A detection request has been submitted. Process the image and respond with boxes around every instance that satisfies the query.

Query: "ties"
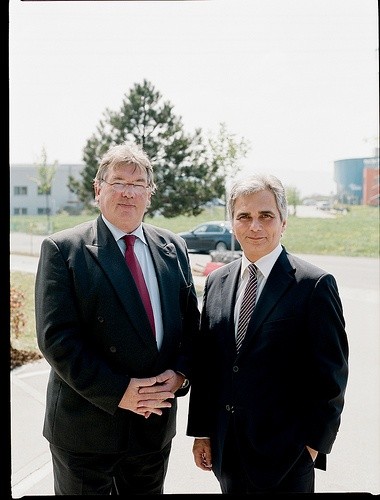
[236,264,258,353]
[120,235,156,337]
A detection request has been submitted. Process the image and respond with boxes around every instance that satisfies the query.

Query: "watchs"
[181,377,190,389]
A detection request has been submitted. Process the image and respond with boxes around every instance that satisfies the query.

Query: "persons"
[193,174,349,493]
[35,145,200,496]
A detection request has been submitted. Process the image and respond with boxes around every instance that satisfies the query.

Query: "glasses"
[98,178,151,192]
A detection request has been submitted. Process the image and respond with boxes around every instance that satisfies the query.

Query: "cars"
[174,221,244,253]
